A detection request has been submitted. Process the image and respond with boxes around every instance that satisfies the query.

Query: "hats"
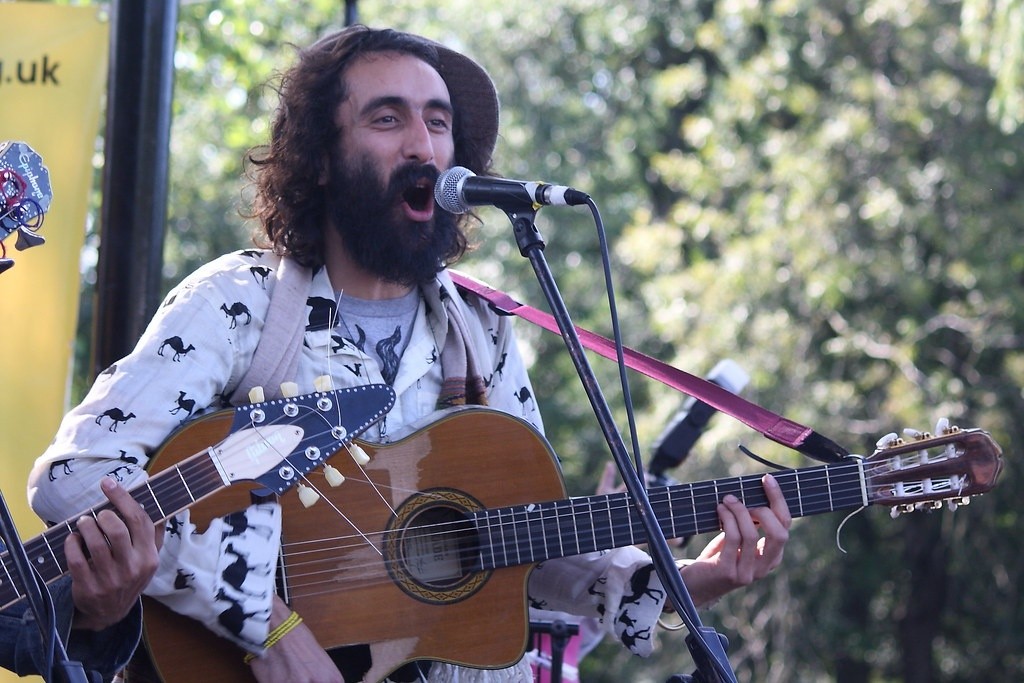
[281,29,498,174]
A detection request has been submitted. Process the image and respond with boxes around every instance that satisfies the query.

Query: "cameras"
[644,359,750,549]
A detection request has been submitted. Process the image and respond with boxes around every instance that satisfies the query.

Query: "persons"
[593,462,684,551]
[27,20,789,683]
[0,473,166,683]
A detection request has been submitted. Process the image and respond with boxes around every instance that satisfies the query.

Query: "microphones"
[434,165,590,215]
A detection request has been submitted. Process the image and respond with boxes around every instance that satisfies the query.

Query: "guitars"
[0,381,398,613]
[140,405,1005,683]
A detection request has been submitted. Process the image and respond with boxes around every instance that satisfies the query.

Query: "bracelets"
[243,611,304,663]
[657,617,686,631]
